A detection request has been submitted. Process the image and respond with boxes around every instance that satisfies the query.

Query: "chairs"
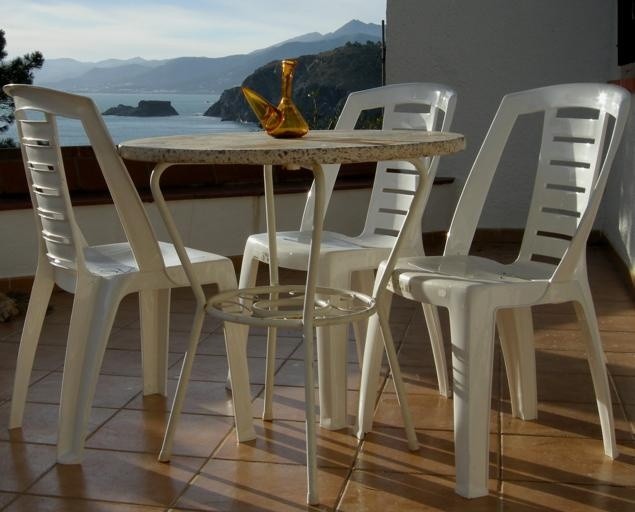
[352,82,633,498]
[224,81,459,429]
[1,82,256,463]
